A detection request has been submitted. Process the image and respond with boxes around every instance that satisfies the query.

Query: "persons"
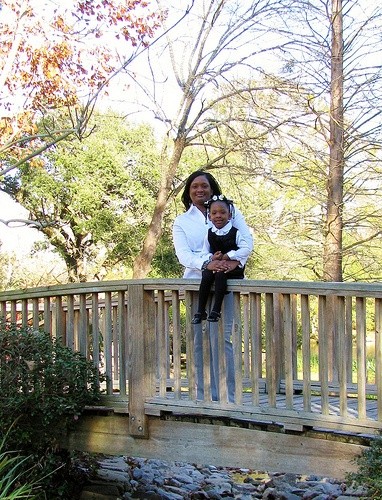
[189,194,250,324]
[173,171,254,403]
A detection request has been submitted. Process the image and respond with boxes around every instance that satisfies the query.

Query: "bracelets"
[202,260,211,269]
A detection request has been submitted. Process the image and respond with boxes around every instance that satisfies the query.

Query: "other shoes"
[190,311,207,324]
[207,310,221,322]
